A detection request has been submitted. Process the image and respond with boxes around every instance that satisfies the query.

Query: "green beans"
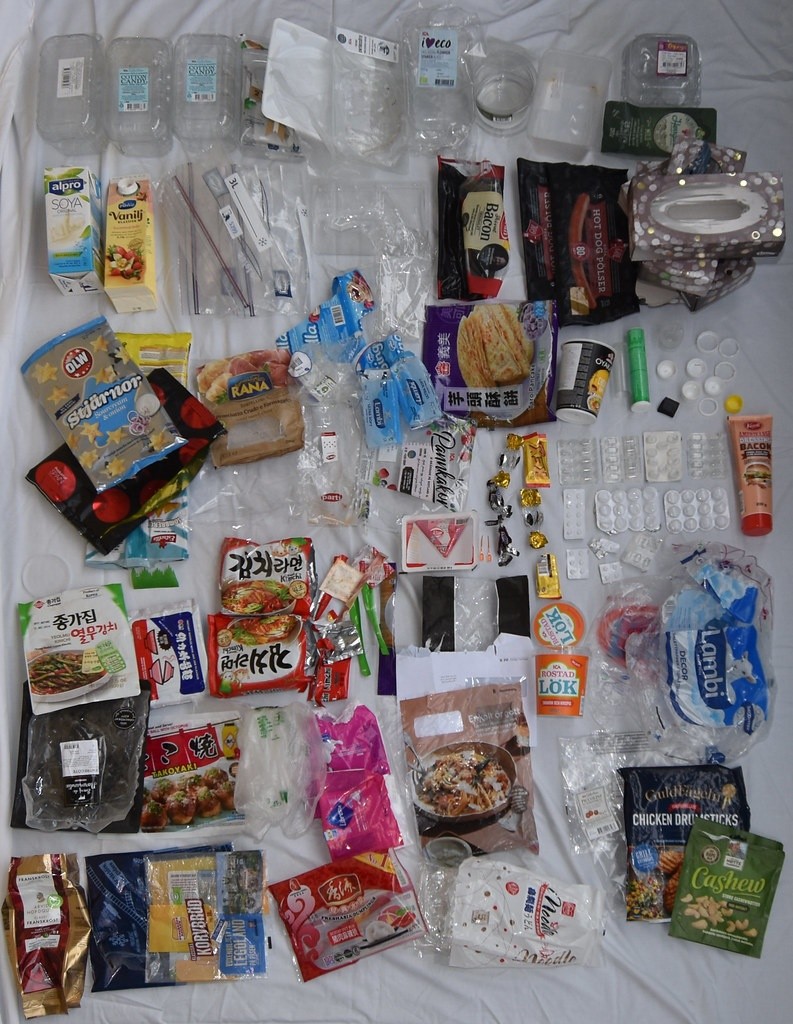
[30,656,94,689]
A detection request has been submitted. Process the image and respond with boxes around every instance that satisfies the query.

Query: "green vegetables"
[232,579,290,644]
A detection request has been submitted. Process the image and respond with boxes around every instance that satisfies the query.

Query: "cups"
[555,338,616,424]
[533,647,590,720]
[473,50,537,132]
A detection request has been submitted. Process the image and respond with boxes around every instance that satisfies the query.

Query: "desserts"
[139,766,238,829]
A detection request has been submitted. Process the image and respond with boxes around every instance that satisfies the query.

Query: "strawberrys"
[108,245,143,276]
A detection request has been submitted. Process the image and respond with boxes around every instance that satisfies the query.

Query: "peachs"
[36,382,221,524]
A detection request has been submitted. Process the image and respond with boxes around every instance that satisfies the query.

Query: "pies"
[457,303,535,389]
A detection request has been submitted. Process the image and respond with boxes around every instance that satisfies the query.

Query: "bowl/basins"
[526,50,612,149]
[101,36,172,156]
[410,741,518,825]
[172,32,240,156]
[36,33,107,155]
[620,33,702,107]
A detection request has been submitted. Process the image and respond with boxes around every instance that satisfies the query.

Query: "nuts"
[681,894,758,937]
